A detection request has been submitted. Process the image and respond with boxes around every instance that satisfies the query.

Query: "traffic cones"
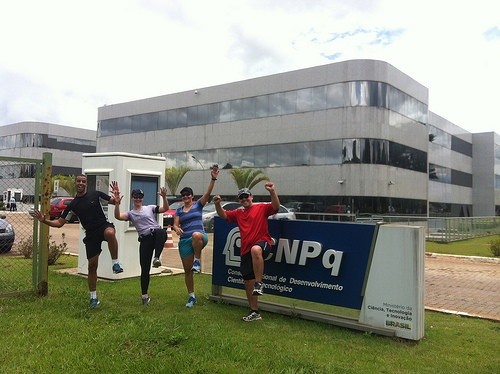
[163,225,174,249]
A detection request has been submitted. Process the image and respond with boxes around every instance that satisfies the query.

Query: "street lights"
[192,156,205,196]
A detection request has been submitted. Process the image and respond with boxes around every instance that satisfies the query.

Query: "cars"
[0,214,15,253]
[164,201,296,234]
[283,202,348,221]
[28,197,79,223]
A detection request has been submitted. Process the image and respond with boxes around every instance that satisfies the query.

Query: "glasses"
[132,195,144,199]
[238,194,249,200]
[180,192,192,197]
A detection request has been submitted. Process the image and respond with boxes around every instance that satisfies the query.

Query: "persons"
[173,167,220,307]
[10,196,16,212]
[213,183,282,322]
[112,187,169,304]
[33,175,124,306]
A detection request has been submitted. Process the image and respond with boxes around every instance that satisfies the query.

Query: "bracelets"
[212,178,217,180]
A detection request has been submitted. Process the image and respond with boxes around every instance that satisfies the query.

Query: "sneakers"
[185,296,197,308]
[90,297,100,310]
[190,260,201,274]
[112,262,124,274]
[152,257,162,269]
[141,296,151,306]
[242,312,262,322]
[251,281,264,296]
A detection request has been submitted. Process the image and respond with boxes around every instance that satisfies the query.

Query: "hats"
[180,186,193,196]
[238,188,252,198]
[131,189,145,198]
